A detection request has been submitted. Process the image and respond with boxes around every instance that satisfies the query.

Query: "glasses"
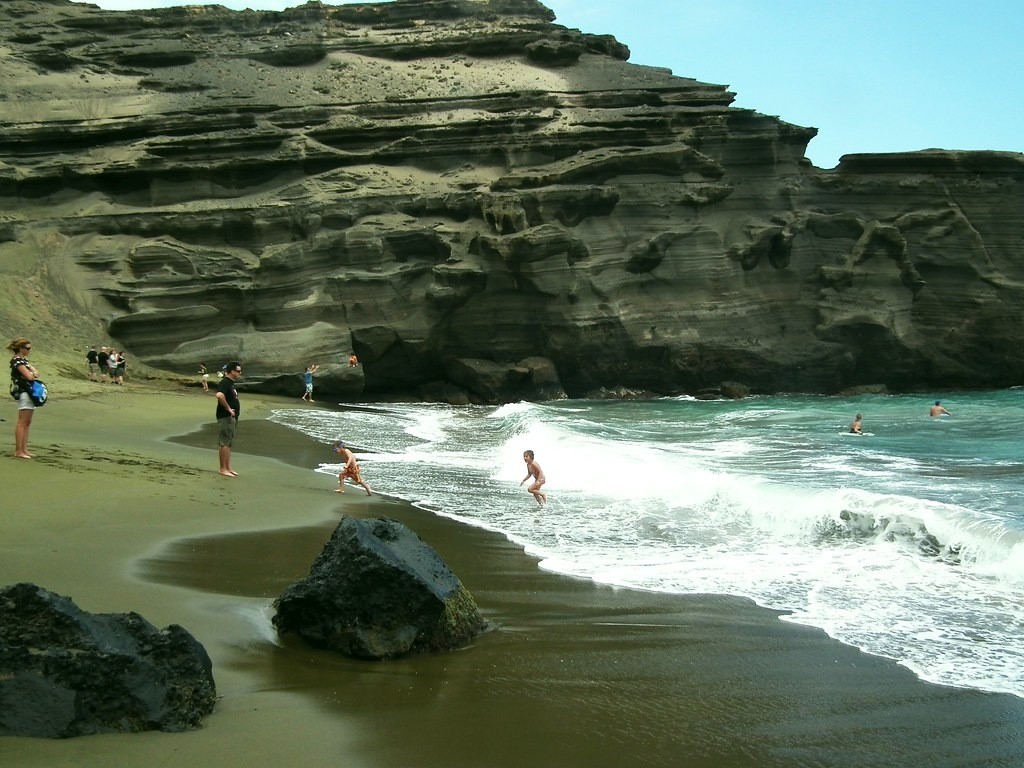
[22,346,31,350]
[234,370,241,373]
[332,448,337,452]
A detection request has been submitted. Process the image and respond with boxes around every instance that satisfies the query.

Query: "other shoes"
[90,378,122,386]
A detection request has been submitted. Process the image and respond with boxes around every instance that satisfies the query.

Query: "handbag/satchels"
[24,379,49,407]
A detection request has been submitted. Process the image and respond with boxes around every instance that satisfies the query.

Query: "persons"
[332,440,371,496]
[98,346,110,383]
[118,351,127,385]
[850,413,864,437]
[518,450,545,506]
[219,365,238,398]
[303,364,319,402]
[198,362,209,392]
[927,400,952,418]
[85,345,100,382]
[6,337,39,460]
[216,362,243,479]
[109,348,118,386]
[347,354,359,367]
